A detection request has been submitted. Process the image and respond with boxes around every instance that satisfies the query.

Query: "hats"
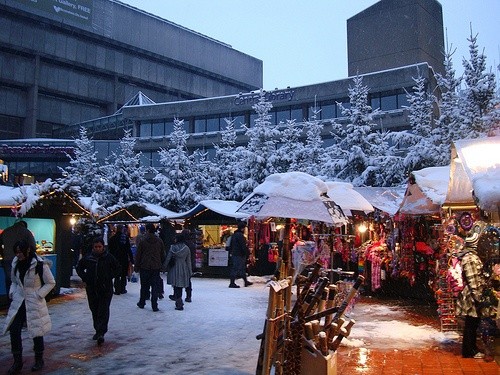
[465,230,479,246]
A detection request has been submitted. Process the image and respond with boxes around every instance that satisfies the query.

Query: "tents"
[0,135,500,226]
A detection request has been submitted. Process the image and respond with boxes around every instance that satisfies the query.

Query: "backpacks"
[445,258,467,298]
[13,260,53,304]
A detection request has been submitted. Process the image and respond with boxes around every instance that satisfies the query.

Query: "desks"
[0,253,59,300]
[203,247,234,276]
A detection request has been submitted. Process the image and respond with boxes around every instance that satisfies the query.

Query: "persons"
[72,225,194,303]
[456,232,487,359]
[76,236,123,346]
[1,239,55,375]
[134,223,165,312]
[0,221,36,308]
[228,223,253,288]
[165,235,193,311]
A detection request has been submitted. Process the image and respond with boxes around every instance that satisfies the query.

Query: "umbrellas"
[235,172,349,277]
[323,182,375,283]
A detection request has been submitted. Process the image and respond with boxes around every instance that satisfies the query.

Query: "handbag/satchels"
[168,256,175,267]
[130,271,139,283]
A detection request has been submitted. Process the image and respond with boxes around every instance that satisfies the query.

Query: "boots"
[185,290,192,302]
[31,351,44,371]
[169,295,176,301]
[8,351,23,374]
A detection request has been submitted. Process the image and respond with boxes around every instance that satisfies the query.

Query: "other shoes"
[474,352,485,359]
[245,281,253,286]
[229,283,240,288]
[96,336,104,345]
